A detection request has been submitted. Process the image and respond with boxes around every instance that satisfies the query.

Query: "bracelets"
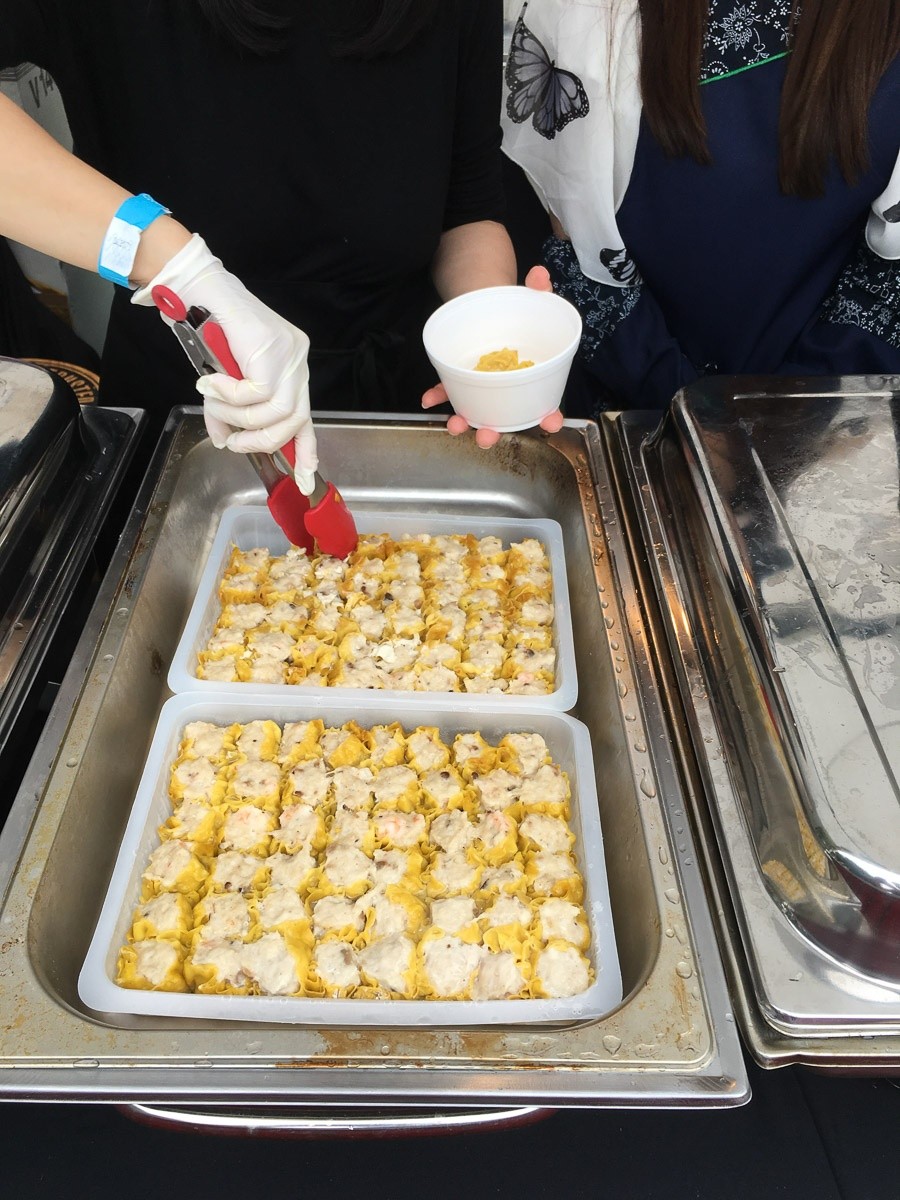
[96,192,171,291]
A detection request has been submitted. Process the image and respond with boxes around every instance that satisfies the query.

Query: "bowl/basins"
[424,285,582,432]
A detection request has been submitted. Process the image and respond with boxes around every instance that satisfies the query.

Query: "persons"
[1,0,564,497]
[492,0,899,413]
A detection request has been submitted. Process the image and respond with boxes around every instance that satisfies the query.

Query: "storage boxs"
[167,506,577,711]
[77,692,622,1024]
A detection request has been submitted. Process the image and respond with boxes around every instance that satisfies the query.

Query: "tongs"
[152,286,361,560]
